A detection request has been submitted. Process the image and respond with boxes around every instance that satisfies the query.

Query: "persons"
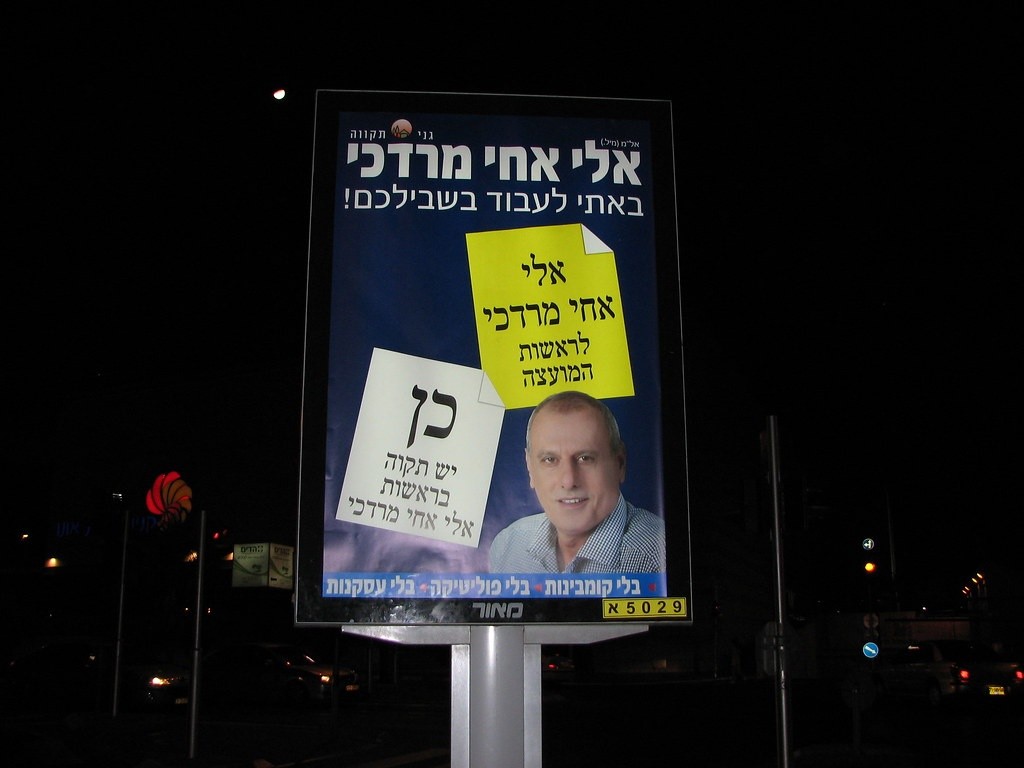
[489,390,666,574]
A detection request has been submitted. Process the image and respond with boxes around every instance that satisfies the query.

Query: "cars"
[843,638,1024,703]
[294,652,361,702]
[542,656,577,682]
[201,643,305,708]
[63,635,191,710]
